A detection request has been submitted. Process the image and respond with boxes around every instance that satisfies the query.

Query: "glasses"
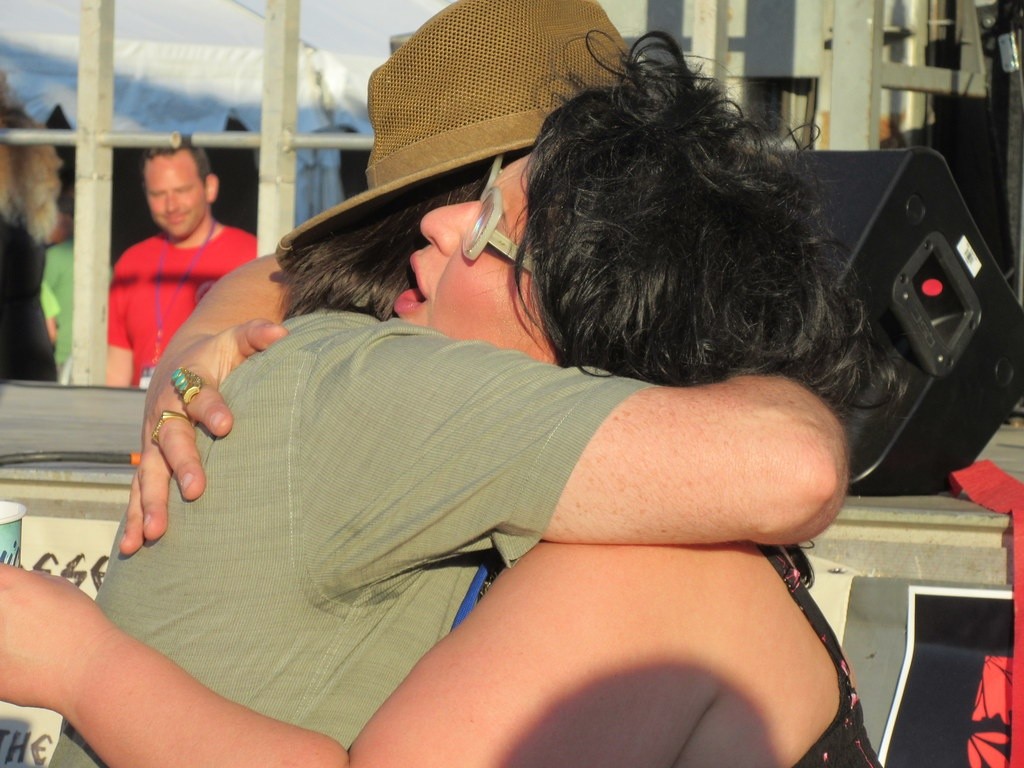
[462,155,541,274]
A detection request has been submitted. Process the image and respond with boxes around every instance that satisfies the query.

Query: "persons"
[1,33,881,767]
[53,0,852,768]
[2,132,258,392]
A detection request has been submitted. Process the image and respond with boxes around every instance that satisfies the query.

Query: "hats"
[275,0,638,272]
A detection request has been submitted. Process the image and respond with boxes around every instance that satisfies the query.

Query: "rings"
[131,452,143,465]
[150,409,191,447]
[170,364,207,407]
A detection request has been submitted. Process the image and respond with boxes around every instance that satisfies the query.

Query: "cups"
[0,501,26,567]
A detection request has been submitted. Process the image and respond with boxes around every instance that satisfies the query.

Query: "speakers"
[761,145,1024,496]
